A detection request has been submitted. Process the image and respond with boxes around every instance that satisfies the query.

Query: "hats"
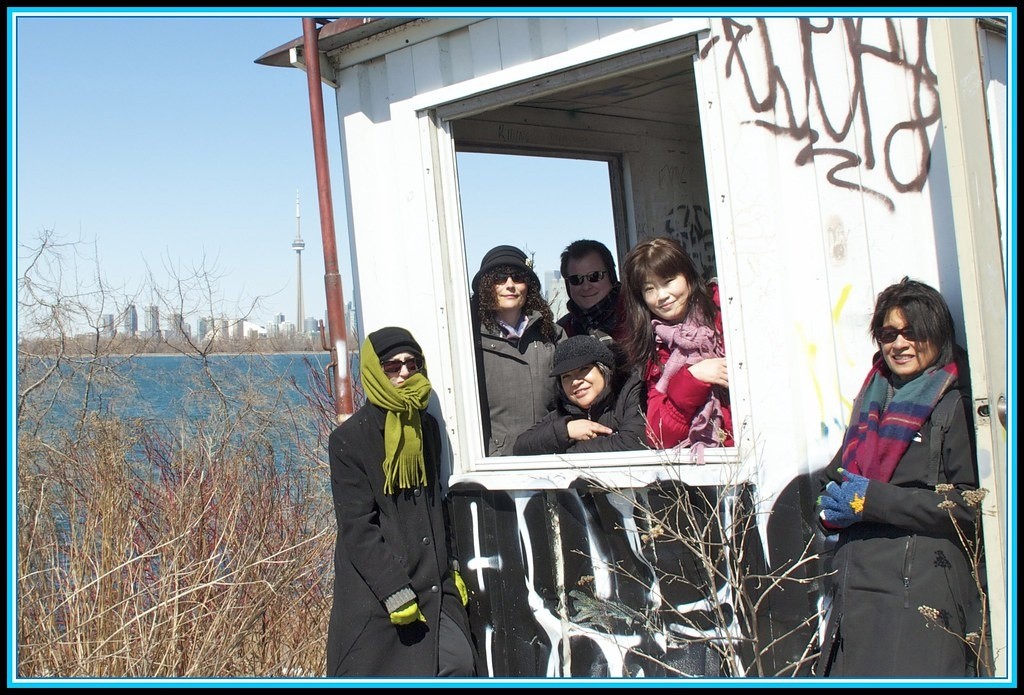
[471,245,541,291]
[548,334,613,378]
[367,327,422,362]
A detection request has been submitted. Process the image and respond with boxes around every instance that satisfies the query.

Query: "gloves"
[390,599,427,625]
[816,468,869,531]
[454,570,469,609]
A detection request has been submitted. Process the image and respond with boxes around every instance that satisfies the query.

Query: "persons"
[513,334,651,456]
[613,234,737,447]
[552,238,647,380]
[468,245,569,457]
[801,273,986,681]
[325,326,488,678]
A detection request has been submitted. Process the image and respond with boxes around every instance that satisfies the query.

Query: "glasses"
[876,324,927,343]
[563,268,610,286]
[380,356,424,373]
[492,270,527,285]
